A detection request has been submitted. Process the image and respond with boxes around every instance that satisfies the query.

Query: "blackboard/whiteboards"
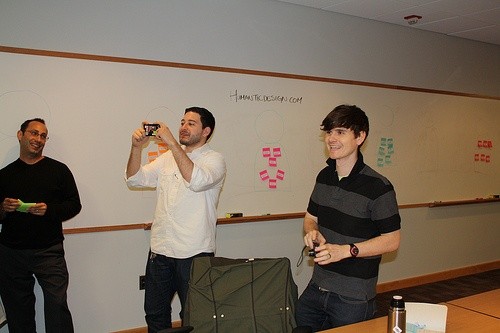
[0,44,496,234]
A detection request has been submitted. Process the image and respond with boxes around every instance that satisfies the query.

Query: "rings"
[35,209,39,213]
[328,254,331,258]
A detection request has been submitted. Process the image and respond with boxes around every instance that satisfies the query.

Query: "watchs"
[349,243,359,258]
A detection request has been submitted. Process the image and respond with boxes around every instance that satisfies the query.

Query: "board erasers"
[226,212,243,218]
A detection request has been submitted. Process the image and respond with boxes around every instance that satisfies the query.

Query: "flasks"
[387,296,406,333]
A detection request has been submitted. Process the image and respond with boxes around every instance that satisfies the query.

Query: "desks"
[316,287,500,333]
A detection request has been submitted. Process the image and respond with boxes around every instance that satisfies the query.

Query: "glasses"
[25,130,49,142]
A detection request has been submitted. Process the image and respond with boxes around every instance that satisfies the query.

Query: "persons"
[0,118,82,333]
[291,104,401,333]
[124,107,227,333]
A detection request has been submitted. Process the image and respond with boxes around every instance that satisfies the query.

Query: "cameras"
[144,123,161,136]
[308,241,320,257]
